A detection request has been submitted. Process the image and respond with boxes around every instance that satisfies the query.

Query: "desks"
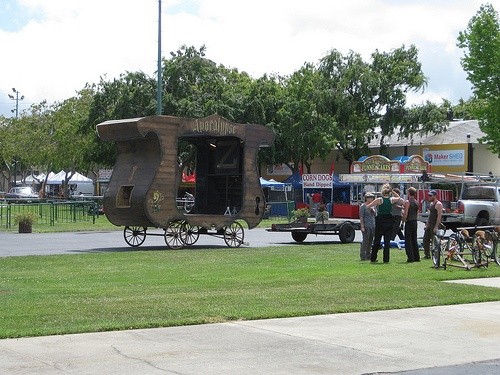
[207,173,242,214]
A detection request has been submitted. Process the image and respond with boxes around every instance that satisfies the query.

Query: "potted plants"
[290,207,310,222]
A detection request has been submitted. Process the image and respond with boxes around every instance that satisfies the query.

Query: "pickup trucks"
[418,184,500,236]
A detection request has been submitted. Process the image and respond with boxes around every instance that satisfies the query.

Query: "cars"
[6,186,40,204]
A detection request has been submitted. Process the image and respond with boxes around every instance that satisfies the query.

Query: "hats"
[364,192,375,198]
[427,190,438,196]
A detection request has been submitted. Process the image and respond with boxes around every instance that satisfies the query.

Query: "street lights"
[8,88,25,186]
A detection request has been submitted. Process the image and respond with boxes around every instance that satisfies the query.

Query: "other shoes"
[359,255,431,263]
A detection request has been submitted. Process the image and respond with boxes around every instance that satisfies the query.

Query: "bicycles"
[431,221,500,270]
